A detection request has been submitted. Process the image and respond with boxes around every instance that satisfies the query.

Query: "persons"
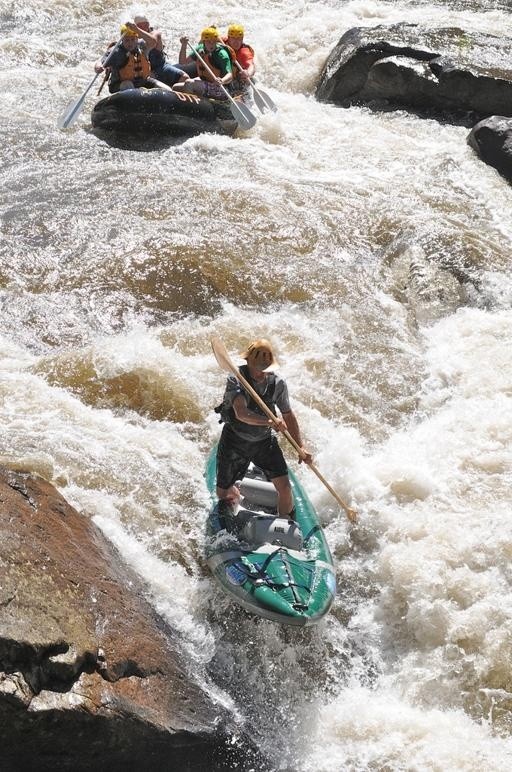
[94,15,255,103]
[216,339,313,523]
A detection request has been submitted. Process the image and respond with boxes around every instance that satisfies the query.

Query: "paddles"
[209,333,358,522]
[59,26,132,130]
[217,37,278,116]
[185,40,257,131]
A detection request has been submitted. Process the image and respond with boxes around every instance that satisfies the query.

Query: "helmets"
[198,28,221,45]
[240,338,280,372]
[121,25,138,37]
[228,25,245,39]
[134,13,148,25]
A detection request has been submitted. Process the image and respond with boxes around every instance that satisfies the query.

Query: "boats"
[202,437,336,627]
[91,85,256,152]
